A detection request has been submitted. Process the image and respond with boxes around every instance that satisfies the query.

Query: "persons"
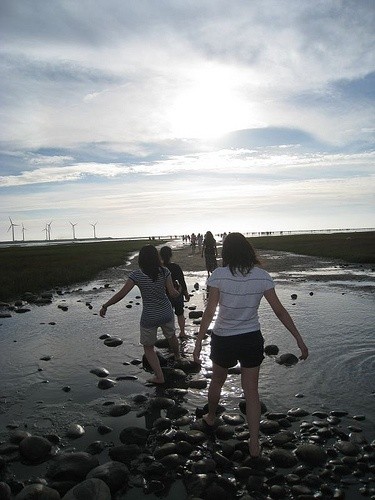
[200,231,219,278]
[191,231,310,460]
[148,231,232,256]
[158,246,191,353]
[99,244,187,386]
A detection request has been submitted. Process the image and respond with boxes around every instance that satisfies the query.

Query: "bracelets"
[102,305,107,309]
[195,343,201,347]
